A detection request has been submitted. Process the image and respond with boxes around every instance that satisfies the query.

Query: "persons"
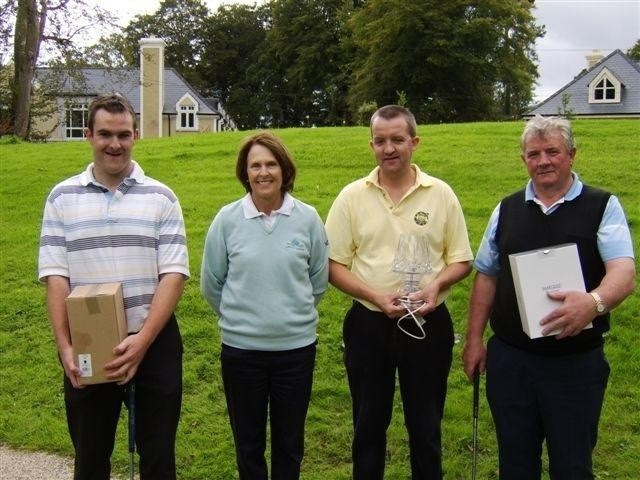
[315,102,473,478]
[196,132,330,479]
[33,90,193,478]
[450,112,638,480]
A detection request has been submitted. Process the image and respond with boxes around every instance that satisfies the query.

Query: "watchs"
[587,290,608,317]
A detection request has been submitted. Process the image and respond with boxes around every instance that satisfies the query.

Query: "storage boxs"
[63,282,129,387]
